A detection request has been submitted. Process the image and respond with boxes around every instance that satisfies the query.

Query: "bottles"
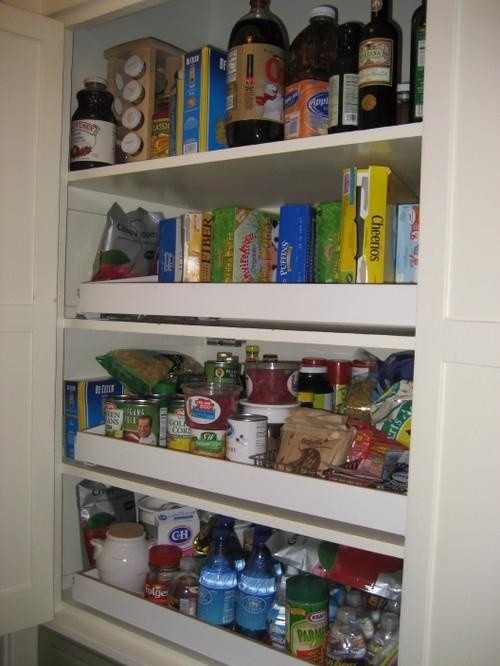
[326,20,365,134]
[357,0,399,131]
[283,5,341,140]
[68,76,118,172]
[408,0,428,124]
[143,513,401,666]
[216,344,376,415]
[225,0,288,148]
[114,56,169,154]
[396,83,411,125]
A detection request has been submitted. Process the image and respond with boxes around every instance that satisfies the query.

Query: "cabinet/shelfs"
[0,0,500,666]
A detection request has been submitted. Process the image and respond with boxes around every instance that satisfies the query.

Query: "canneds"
[225,415,268,465]
[149,393,171,447]
[204,360,238,389]
[122,400,160,443]
[166,397,192,451]
[103,392,134,438]
